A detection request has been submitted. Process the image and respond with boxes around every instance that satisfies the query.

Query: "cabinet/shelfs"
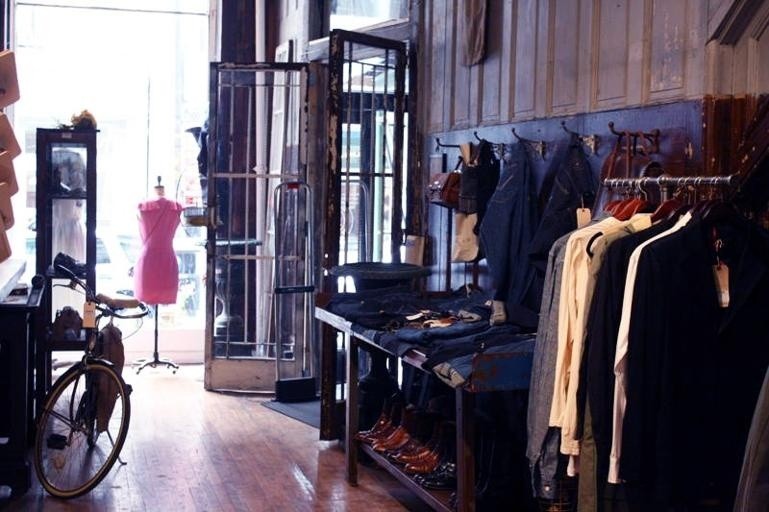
[314,305,475,512]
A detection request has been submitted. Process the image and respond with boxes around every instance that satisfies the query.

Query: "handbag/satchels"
[54,307,82,341]
[589,151,665,222]
[451,213,479,262]
[427,173,460,208]
[458,160,500,213]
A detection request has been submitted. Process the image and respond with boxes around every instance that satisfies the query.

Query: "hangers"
[603,175,754,235]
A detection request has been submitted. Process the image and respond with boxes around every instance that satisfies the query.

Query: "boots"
[354,390,497,512]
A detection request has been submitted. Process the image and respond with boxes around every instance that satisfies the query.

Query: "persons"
[52,150,87,264]
[135,185,186,305]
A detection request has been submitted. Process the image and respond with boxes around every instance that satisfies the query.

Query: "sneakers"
[54,253,87,277]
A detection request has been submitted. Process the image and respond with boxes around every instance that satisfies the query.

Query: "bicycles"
[35,264,151,501]
